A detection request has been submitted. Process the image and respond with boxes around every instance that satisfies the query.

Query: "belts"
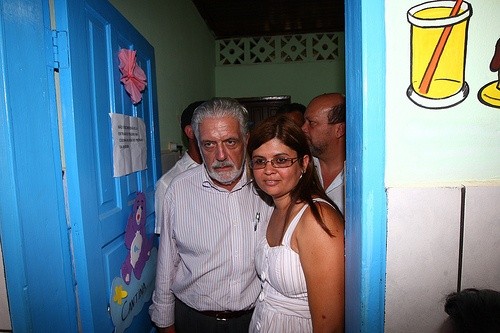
[193,308,254,320]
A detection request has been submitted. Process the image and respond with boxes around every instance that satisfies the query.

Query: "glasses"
[248,156,299,168]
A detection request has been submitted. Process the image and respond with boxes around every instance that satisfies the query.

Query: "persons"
[246,115,345,333]
[300,93,346,218]
[273,103,307,127]
[154,101,206,234]
[146,96,275,333]
